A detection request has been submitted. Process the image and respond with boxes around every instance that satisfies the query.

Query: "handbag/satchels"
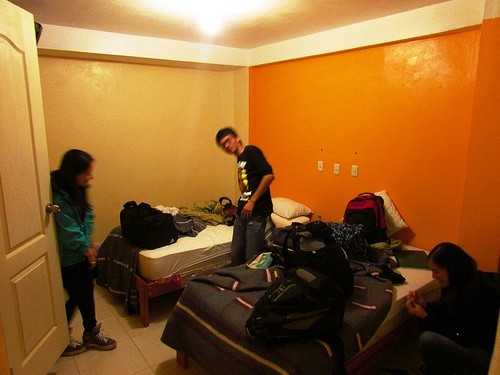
[219,197,238,225]
[282,221,372,301]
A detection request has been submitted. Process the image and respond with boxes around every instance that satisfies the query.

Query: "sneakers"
[60,327,86,356]
[83,322,117,350]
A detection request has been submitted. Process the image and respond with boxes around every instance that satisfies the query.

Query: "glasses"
[220,136,234,149]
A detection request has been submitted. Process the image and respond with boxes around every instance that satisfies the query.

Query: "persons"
[406,241,500,375]
[214,127,277,270]
[49,148,117,357]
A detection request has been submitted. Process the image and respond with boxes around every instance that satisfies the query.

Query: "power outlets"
[334,164,339,174]
[317,161,323,171]
[352,165,359,176]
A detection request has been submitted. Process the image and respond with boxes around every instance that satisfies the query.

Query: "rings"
[90,261,95,264]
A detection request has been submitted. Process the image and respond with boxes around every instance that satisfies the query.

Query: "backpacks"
[120,201,178,248]
[244,264,345,346]
[343,193,388,243]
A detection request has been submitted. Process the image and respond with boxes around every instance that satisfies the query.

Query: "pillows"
[341,190,407,237]
[271,196,311,227]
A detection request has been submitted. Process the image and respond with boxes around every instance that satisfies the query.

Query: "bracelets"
[250,198,255,203]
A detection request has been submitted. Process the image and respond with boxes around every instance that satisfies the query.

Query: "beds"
[133,225,442,375]
